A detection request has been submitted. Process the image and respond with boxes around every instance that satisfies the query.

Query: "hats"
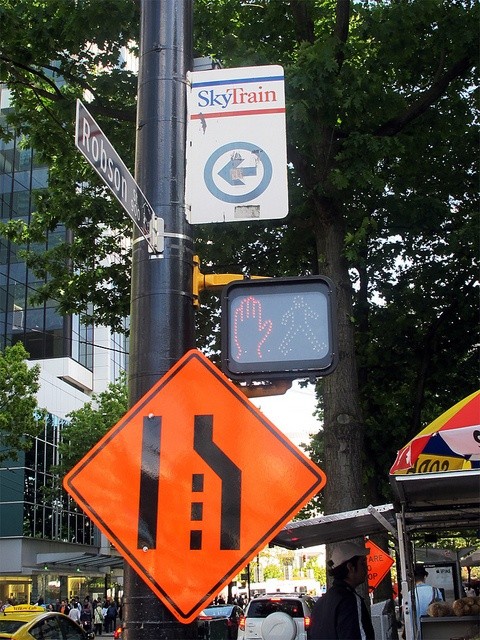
[414,564,428,578]
[329,542,370,570]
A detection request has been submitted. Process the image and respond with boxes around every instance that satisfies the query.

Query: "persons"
[34,599,46,610]
[94,603,102,634]
[97,597,101,603]
[54,599,60,612]
[209,591,251,606]
[70,599,81,611]
[409,565,443,639]
[74,596,81,603]
[102,603,109,632]
[82,603,92,632]
[58,601,69,614]
[308,539,375,639]
[47,604,54,611]
[68,604,80,624]
[107,603,117,632]
[84,599,90,603]
[65,600,70,609]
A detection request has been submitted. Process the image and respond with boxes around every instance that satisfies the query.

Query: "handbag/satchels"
[96,607,103,622]
[428,586,442,606]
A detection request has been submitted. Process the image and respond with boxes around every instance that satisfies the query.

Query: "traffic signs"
[74,99,162,253]
[184,65,289,224]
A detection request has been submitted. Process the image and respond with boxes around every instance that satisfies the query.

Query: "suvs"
[237,593,316,640]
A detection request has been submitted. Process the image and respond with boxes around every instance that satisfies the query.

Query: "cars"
[0,604,95,640]
[198,604,245,640]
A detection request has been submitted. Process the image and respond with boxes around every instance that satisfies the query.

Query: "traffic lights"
[222,275,339,380]
